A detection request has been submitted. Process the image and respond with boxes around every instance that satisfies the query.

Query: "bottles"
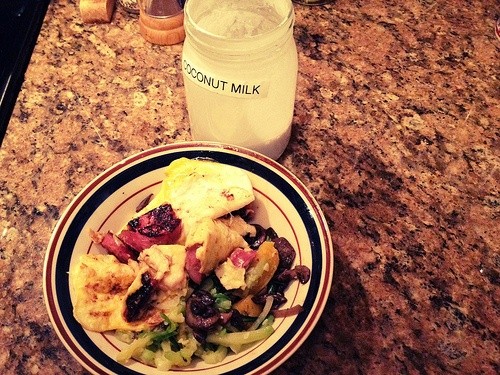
[180,0,299,161]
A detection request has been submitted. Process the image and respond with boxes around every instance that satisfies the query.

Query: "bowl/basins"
[42,140,336,375]
